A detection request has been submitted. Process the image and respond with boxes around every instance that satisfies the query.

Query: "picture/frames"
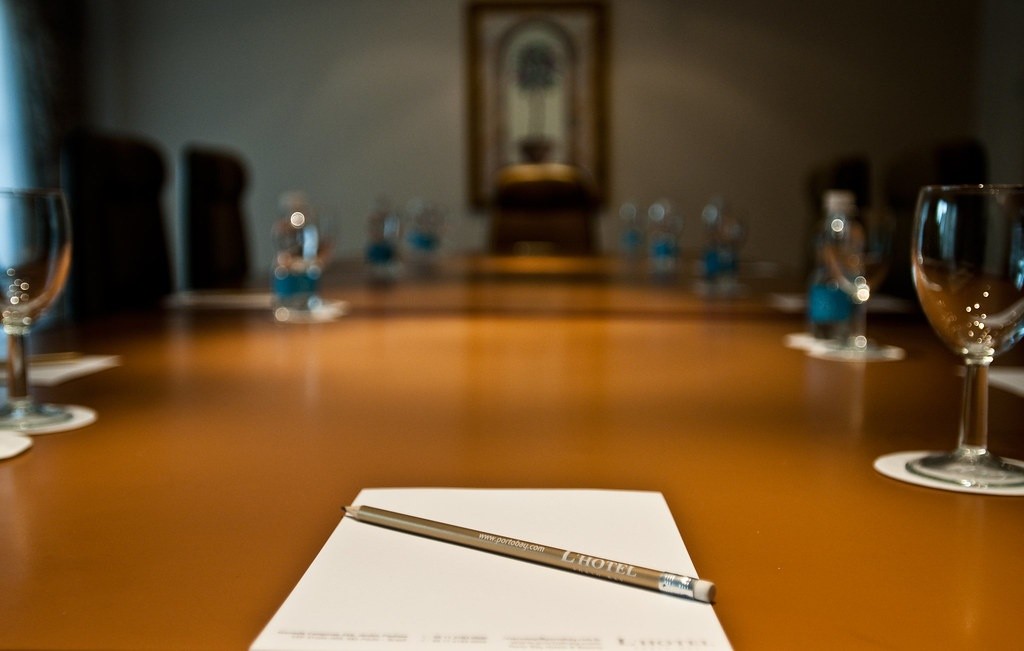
[459,0,618,212]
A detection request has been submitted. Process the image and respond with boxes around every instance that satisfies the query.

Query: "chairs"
[45,126,178,323]
[182,139,254,294]
[798,129,993,328]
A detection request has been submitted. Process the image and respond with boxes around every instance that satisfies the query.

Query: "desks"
[0,253,1024,651]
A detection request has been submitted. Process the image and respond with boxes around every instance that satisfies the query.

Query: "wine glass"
[908,181,1024,488]
[0,185,73,429]
[818,198,906,363]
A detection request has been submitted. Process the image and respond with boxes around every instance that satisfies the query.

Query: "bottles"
[808,189,862,340]
[365,188,402,288]
[270,187,320,318]
[409,201,442,276]
[621,195,741,297]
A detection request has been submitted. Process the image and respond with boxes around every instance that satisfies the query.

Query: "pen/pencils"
[339,504,717,603]
